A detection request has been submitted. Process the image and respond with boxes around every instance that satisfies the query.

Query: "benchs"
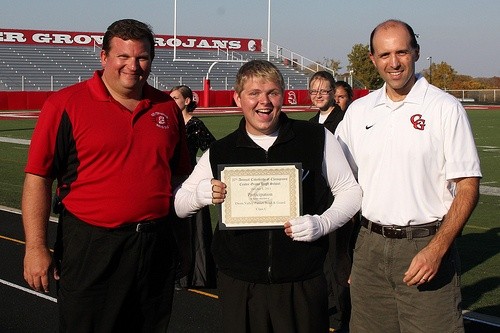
[0,43,310,91]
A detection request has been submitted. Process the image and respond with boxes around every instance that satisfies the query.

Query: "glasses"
[309,89,332,95]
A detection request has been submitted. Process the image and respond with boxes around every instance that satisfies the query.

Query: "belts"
[118,215,174,232]
[360,214,443,239]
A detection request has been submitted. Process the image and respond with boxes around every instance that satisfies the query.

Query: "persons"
[169,85,217,290]
[334,80,353,111]
[308,70,344,135]
[173,59,363,333]
[333,19,500,333]
[21,19,186,333]
